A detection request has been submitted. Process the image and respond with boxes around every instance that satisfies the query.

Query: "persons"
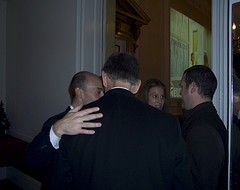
[25,70,105,190]
[61,52,196,190]
[179,65,229,190]
[137,78,167,113]
[230,74,240,190]
